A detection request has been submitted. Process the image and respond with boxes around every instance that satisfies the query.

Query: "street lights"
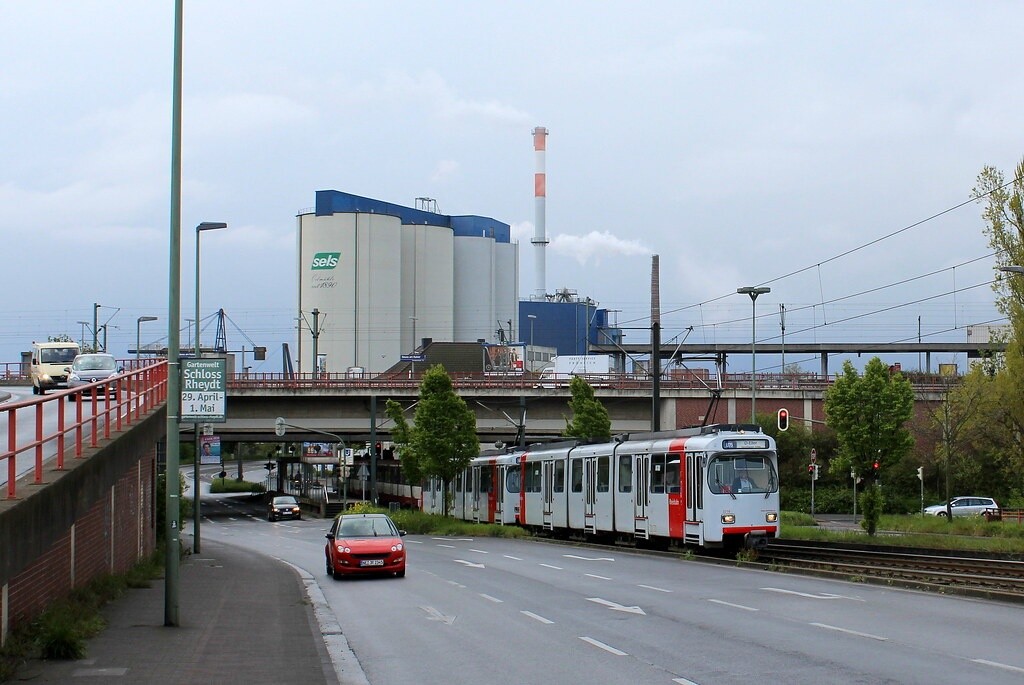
[737,286,771,426]
[193,221,228,550]
[527,314,536,370]
[136,316,158,358]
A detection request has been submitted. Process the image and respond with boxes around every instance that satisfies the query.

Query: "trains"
[349,423,782,555]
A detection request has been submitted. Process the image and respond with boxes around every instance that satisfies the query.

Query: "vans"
[30,342,81,395]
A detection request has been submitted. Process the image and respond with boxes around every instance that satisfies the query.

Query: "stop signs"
[810,447,817,463]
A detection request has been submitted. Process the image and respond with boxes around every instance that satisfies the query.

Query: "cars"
[267,496,302,522]
[920,496,1000,518]
[64,353,125,402]
[325,513,407,580]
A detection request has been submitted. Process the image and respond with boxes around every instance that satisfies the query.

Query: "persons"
[511,348,517,362]
[295,471,302,480]
[314,444,321,455]
[732,470,757,493]
[203,443,213,456]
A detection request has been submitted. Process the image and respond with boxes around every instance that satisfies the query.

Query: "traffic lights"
[779,410,787,429]
[272,463,276,470]
[916,468,922,480]
[376,443,381,454]
[809,465,814,476]
[874,462,879,468]
[264,463,270,470]
